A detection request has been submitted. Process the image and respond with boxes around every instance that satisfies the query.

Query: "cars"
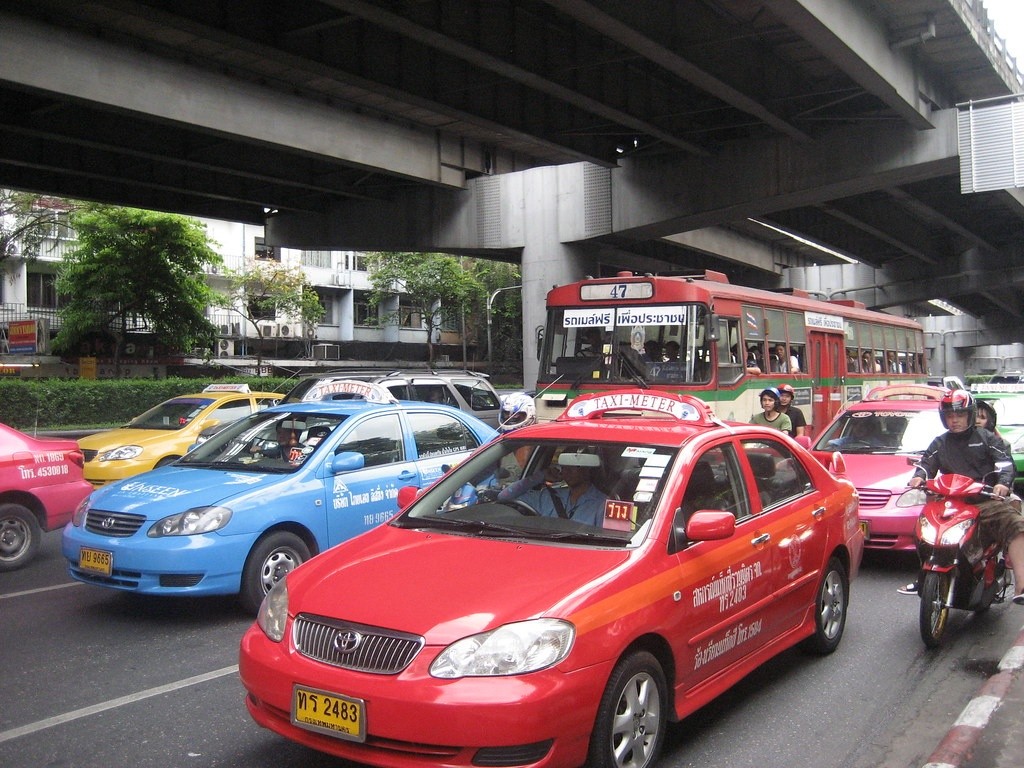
[232,388,867,768]
[57,399,519,619]
[800,371,1024,558]
[0,422,95,574]
[72,380,302,492]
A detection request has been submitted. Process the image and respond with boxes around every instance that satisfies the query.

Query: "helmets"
[777,383,794,400]
[938,389,976,429]
[974,399,997,431]
[498,392,536,431]
[763,387,781,402]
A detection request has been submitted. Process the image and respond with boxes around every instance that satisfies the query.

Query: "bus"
[534,267,931,462]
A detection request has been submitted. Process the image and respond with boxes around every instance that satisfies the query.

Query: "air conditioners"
[217,324,234,336]
[260,324,277,337]
[277,322,295,337]
[5,238,24,258]
[214,338,234,357]
[333,274,351,285]
[311,344,340,360]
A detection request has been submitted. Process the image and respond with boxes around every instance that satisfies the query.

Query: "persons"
[663,340,681,362]
[775,343,801,373]
[862,351,881,373]
[846,349,856,372]
[580,327,609,357]
[749,345,764,372]
[775,383,808,439]
[450,395,561,487]
[888,351,902,373]
[732,342,761,375]
[683,347,708,382]
[912,353,922,372]
[748,387,792,435]
[825,416,885,449]
[249,418,306,462]
[498,445,609,527]
[790,349,797,357]
[897,389,1024,606]
[644,341,662,361]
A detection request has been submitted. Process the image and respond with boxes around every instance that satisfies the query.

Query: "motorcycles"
[904,455,1014,648]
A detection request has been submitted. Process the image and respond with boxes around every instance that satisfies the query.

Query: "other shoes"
[1003,551,1012,568]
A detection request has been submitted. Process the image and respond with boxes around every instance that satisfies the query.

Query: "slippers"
[896,581,919,594]
[1012,588,1024,605]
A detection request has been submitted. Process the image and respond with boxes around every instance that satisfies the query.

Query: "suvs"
[185,367,507,469]
